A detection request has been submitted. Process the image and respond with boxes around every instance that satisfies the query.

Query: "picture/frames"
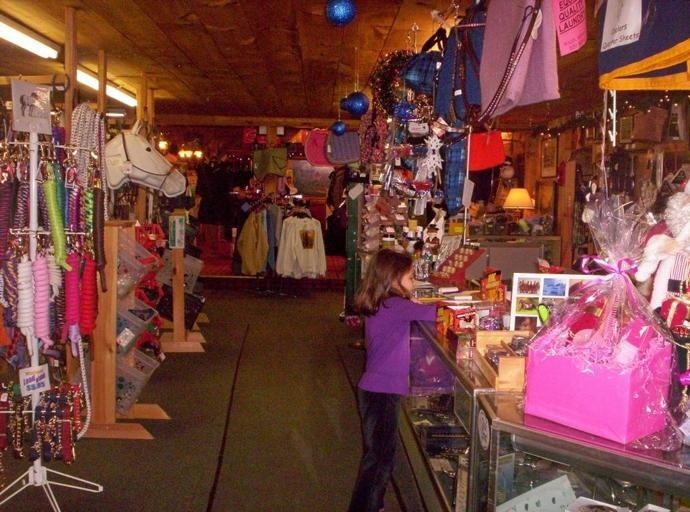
[469,425,471,427]
[535,180,556,231]
[540,136,558,178]
[618,116,634,143]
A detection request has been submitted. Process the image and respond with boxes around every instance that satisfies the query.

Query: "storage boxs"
[116,295,158,354]
[525,341,677,446]
[116,347,161,416]
[117,228,159,300]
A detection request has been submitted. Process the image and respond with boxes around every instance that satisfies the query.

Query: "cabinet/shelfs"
[397,289,690,512]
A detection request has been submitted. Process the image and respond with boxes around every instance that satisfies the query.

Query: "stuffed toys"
[632,193,690,405]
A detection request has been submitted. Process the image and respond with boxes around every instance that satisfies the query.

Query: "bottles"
[412,242,438,282]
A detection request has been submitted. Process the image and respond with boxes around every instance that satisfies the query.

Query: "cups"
[381,234,419,254]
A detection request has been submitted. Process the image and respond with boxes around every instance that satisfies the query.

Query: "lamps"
[105,109,126,117]
[76,64,137,107]
[0,14,62,59]
[502,188,537,213]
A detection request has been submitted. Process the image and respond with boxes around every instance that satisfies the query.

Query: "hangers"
[292,209,312,219]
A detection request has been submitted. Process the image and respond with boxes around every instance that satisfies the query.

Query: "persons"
[345,248,438,511]
